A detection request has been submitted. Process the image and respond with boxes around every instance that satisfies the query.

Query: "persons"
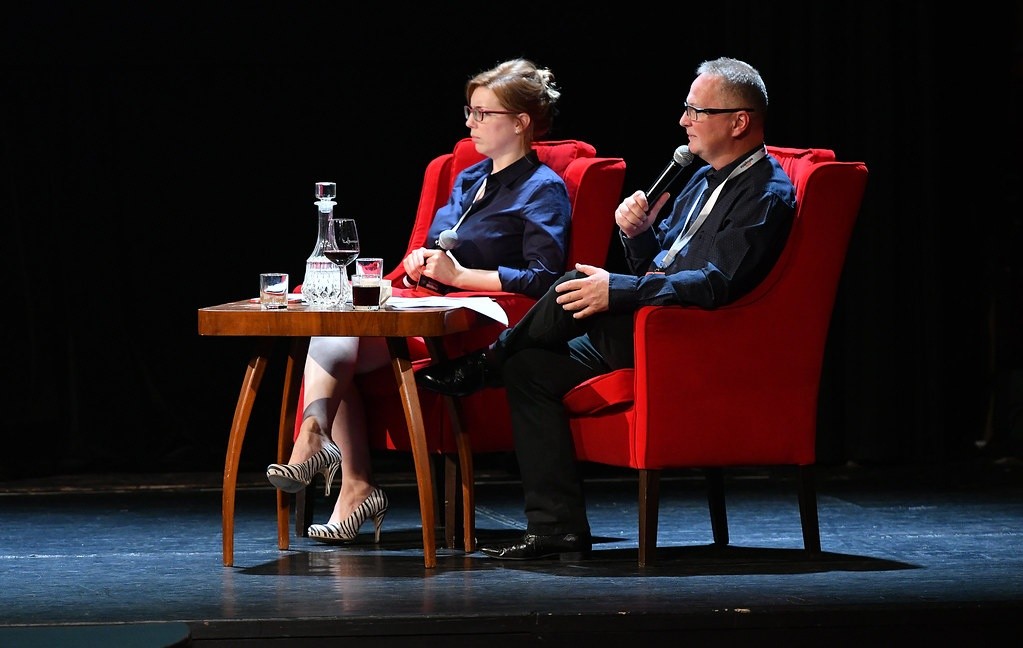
[414,57,801,562]
[267,58,572,542]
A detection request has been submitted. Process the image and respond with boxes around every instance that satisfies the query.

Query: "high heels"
[308,484,388,544]
[267,441,343,497]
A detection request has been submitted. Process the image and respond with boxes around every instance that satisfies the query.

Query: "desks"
[199,293,495,566]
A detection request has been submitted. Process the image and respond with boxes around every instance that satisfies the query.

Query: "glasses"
[684,101,755,122]
[464,105,522,122]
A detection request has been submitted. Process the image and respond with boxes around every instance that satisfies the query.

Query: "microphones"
[402,230,459,289]
[642,144,694,215]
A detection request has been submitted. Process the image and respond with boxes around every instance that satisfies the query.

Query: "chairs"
[292,140,624,550]
[467,144,867,569]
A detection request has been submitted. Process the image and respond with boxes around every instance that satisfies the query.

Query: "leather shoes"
[423,350,507,395]
[480,527,594,561]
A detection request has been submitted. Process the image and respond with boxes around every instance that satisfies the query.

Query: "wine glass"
[323,219,360,310]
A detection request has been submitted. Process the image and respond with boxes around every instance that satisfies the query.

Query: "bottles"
[301,182,350,307]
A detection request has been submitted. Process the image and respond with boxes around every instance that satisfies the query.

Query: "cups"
[352,274,381,310]
[260,273,288,310]
[356,258,383,281]
